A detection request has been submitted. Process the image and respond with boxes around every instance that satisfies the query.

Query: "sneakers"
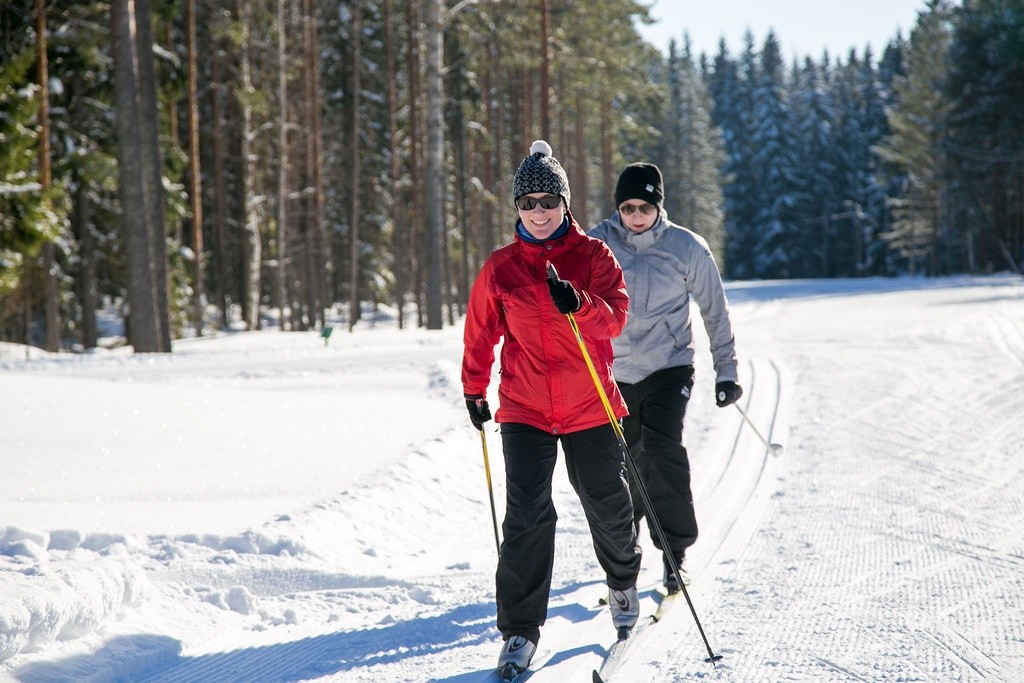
[663,556,693,590]
[497,635,537,679]
[609,584,640,630]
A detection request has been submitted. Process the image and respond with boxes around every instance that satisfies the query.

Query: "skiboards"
[581,575,686,621]
[477,614,651,683]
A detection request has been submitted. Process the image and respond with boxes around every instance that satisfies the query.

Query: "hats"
[512,140,571,210]
[614,161,664,213]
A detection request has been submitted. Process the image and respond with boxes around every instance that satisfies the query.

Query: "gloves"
[546,277,581,314]
[716,381,742,407]
[466,398,492,431]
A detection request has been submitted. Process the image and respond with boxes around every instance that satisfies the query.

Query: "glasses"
[619,203,658,216]
[515,194,562,210]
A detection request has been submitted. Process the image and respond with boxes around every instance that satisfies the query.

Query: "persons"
[586,163,743,592]
[460,141,642,675]
